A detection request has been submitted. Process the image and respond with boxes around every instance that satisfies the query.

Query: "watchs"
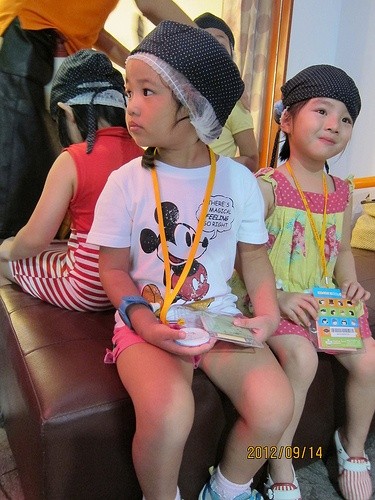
[117,295,154,331]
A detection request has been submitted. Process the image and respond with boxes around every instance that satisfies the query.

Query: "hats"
[270,64,361,174]
[50,49,127,154]
[129,19,245,127]
[193,12,234,51]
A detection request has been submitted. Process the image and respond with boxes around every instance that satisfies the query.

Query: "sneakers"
[264,461,302,500]
[334,426,372,500]
[197,466,264,500]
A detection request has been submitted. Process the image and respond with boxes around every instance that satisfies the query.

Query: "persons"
[256,63,375,500]
[0,48,146,314]
[85,21,296,500]
[195,12,259,171]
[0,0,200,246]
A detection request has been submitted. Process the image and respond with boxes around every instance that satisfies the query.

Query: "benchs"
[0,213,375,500]
